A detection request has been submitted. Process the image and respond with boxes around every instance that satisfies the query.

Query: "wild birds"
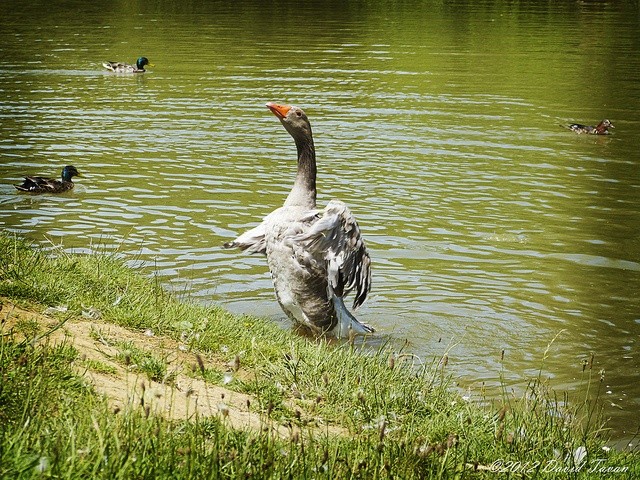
[219,102,378,346]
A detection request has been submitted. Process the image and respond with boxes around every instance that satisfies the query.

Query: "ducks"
[559,119,616,135]
[12,165,87,194]
[102,57,155,73]
